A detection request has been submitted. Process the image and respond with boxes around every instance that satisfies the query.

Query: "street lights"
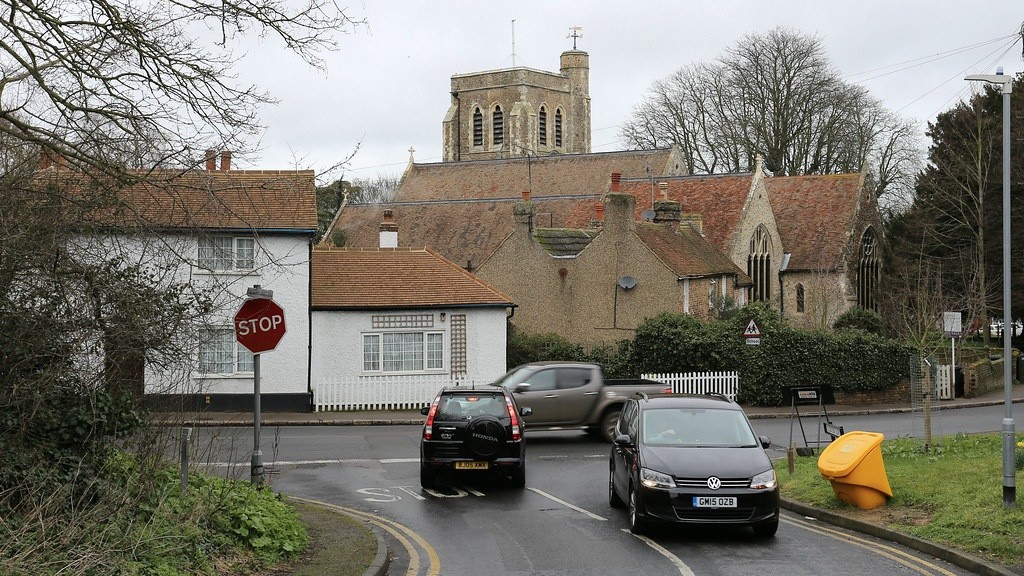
[963,75,1016,514]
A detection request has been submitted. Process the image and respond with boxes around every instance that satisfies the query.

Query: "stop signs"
[233,298,286,355]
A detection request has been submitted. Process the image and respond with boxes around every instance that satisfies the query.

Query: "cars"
[978,323,1023,335]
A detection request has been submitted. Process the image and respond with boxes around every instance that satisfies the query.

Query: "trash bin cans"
[1017,354,1024,382]
[816,431,894,511]
[949,364,962,397]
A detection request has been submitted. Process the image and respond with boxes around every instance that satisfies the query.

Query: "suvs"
[420,383,533,489]
[609,392,780,538]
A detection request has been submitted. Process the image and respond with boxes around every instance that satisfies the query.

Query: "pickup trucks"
[469,361,672,443]
